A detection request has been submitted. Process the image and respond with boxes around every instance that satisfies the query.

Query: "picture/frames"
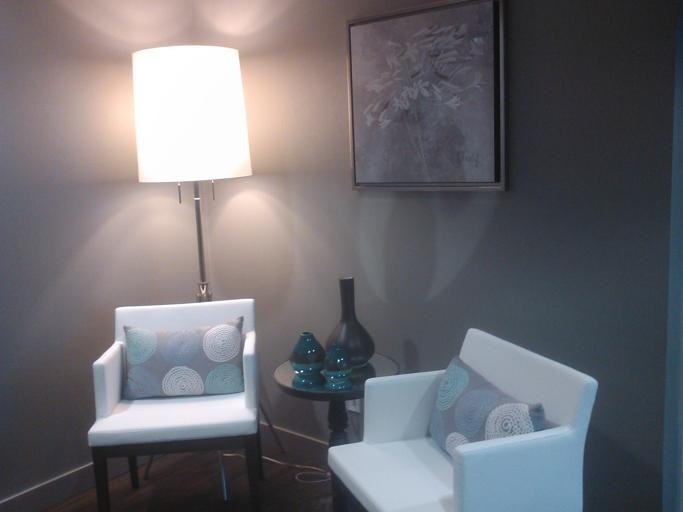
[343,0,509,194]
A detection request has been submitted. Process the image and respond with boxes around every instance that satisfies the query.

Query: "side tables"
[273,351,401,512]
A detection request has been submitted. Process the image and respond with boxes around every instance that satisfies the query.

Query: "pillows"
[118,315,248,402]
[425,356,547,472]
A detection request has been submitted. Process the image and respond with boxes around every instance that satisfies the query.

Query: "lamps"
[132,45,284,497]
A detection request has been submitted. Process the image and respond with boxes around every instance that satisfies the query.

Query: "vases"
[290,276,376,389]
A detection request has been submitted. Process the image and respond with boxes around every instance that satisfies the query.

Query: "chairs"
[327,327,600,512]
[89,298,263,510]
